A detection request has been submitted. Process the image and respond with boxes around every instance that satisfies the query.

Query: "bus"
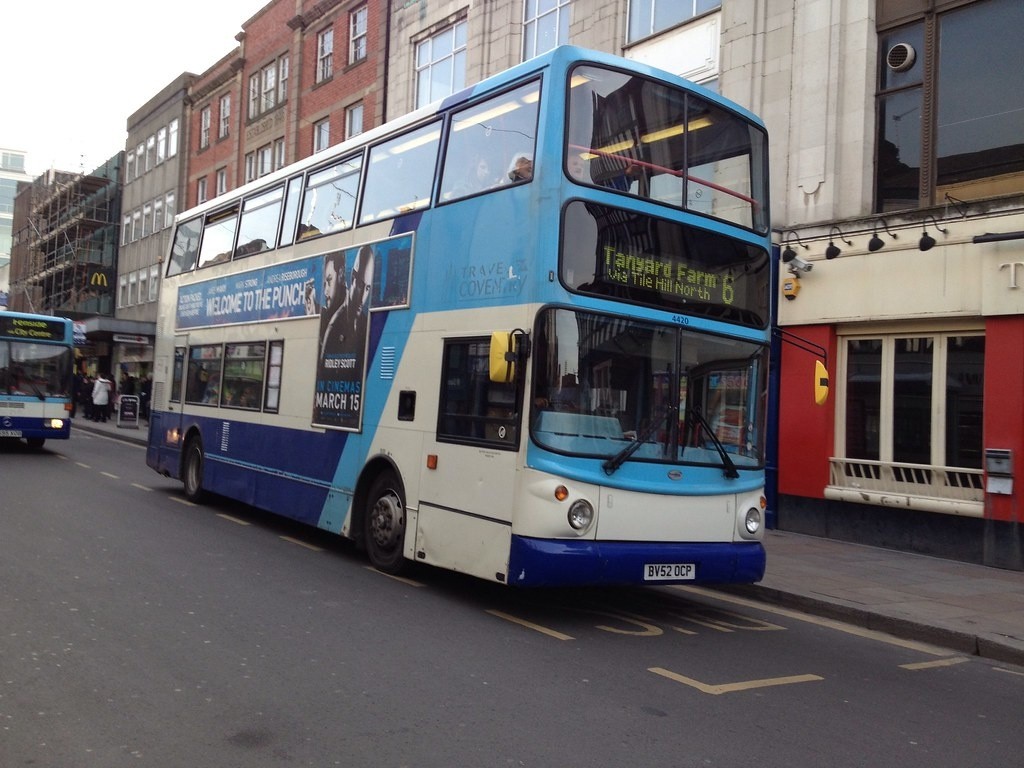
[145,44,780,600]
[0,311,83,450]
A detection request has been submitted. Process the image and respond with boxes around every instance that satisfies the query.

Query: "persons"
[303,250,346,350]
[449,151,642,199]
[321,246,375,381]
[73,372,115,423]
[119,372,152,426]
